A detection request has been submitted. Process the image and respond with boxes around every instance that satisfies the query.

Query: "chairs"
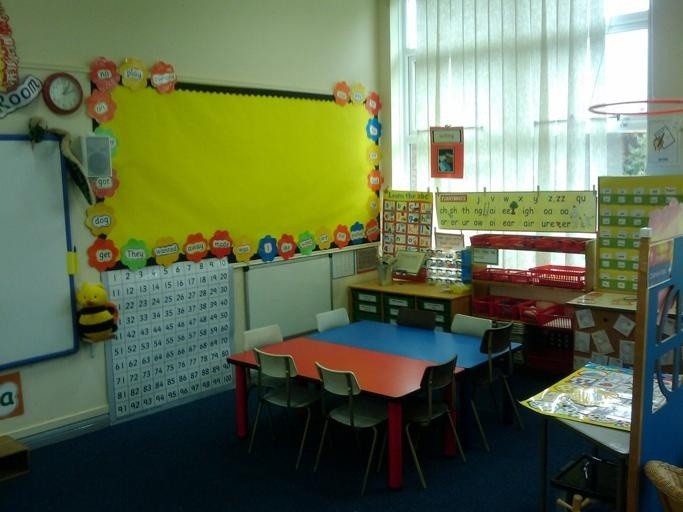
[645,457,683,512]
[464,321,527,458]
[244,346,321,470]
[312,359,388,495]
[240,323,284,430]
[450,313,493,340]
[374,353,470,490]
[314,307,350,333]
[395,308,435,332]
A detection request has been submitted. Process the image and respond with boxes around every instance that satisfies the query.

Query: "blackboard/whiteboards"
[1,133,79,370]
[242,254,335,343]
[94,74,383,269]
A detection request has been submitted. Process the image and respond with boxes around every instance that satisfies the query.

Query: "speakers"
[80,135,113,178]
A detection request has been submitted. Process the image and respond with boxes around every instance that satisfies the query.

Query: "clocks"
[40,69,86,116]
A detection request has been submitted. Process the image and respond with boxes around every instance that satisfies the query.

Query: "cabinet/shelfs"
[347,276,471,335]
[565,292,678,378]
[467,237,598,378]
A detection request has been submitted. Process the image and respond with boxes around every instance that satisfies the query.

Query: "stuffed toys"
[77,280,119,343]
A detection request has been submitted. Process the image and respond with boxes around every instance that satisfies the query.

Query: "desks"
[304,318,523,455]
[225,334,465,489]
[540,410,629,511]
